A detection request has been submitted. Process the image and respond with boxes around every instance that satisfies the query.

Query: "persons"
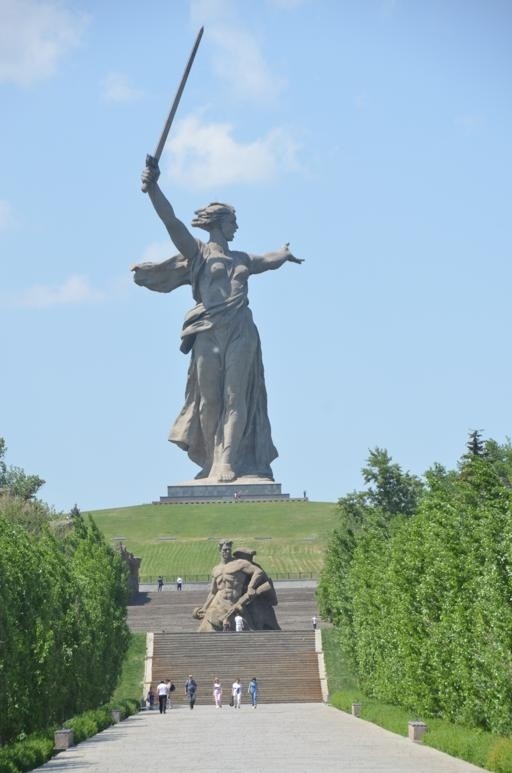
[134,153,306,482]
[193,536,267,631]
[166,677,175,709]
[248,677,257,709]
[311,615,317,628]
[157,679,167,713]
[231,677,243,709]
[148,686,155,706]
[157,575,185,592]
[184,674,198,709]
[212,678,223,708]
